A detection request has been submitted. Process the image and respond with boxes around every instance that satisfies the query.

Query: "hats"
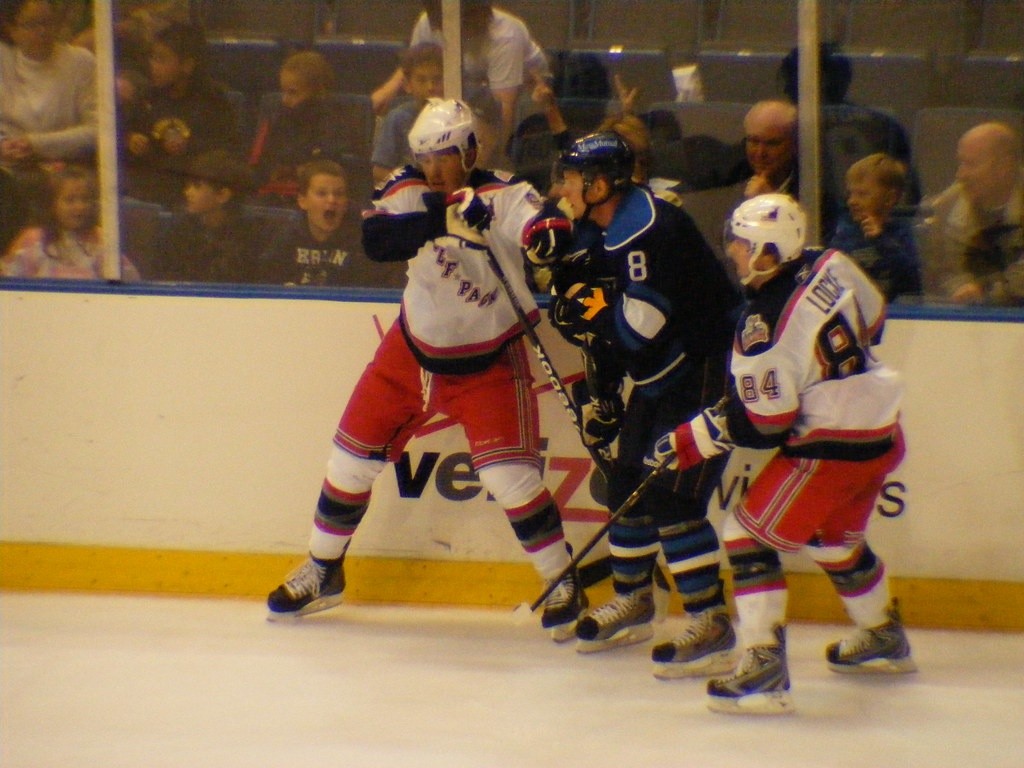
[179,149,249,184]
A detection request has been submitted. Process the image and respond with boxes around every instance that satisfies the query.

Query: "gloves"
[547,260,613,347]
[428,188,490,251]
[644,408,728,472]
[520,212,572,265]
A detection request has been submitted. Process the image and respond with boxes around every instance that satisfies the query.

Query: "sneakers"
[651,602,737,680]
[540,541,588,643]
[824,597,918,674]
[574,584,656,652]
[266,538,351,623]
[705,622,794,714]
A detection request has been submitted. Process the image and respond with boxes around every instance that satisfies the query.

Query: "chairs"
[118,36,1024,290]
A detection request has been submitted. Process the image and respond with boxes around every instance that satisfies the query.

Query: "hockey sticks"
[578,340,615,587]
[482,228,672,627]
[508,384,730,624]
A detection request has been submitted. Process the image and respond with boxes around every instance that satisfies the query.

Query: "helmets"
[553,129,635,205]
[722,190,808,286]
[407,95,480,172]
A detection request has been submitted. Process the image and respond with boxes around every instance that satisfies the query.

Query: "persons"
[266,94,588,627]
[523,130,745,663]
[0,0,1024,308]
[645,194,910,697]
